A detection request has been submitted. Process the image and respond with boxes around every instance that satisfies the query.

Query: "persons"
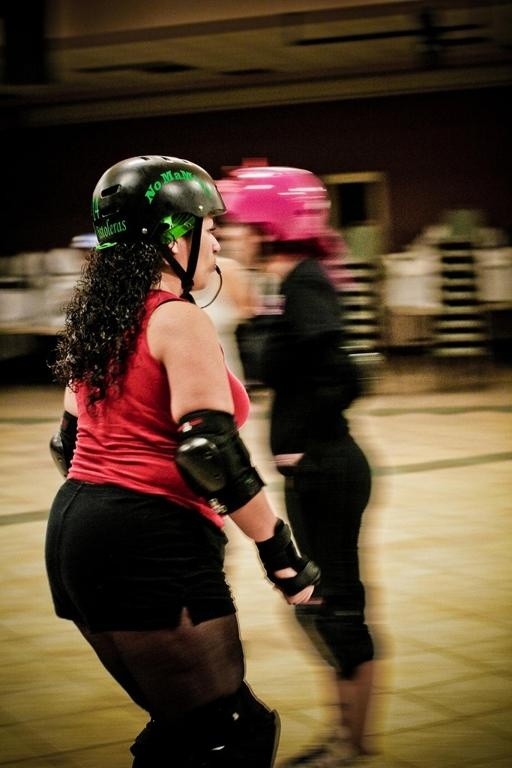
[50,154,324,768]
[213,166,373,768]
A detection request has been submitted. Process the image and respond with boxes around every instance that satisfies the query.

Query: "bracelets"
[255,521,299,571]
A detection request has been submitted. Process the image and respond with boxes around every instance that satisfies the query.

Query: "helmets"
[89,152,230,251]
[225,161,340,244]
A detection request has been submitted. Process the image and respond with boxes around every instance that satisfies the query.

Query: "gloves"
[254,515,323,598]
